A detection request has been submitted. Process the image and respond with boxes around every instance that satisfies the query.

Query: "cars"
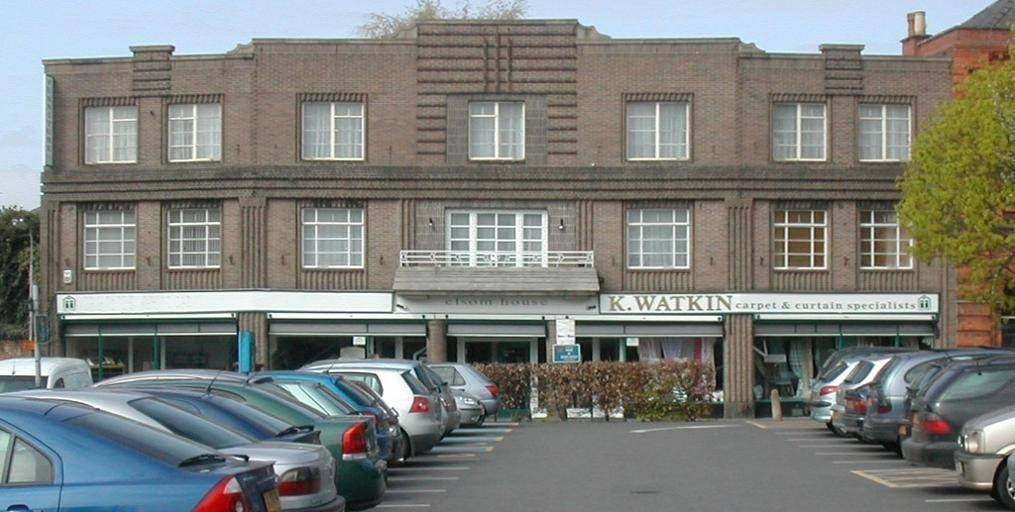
[811,346,1015,510]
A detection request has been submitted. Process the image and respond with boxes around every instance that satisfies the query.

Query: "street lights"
[11,216,33,341]
